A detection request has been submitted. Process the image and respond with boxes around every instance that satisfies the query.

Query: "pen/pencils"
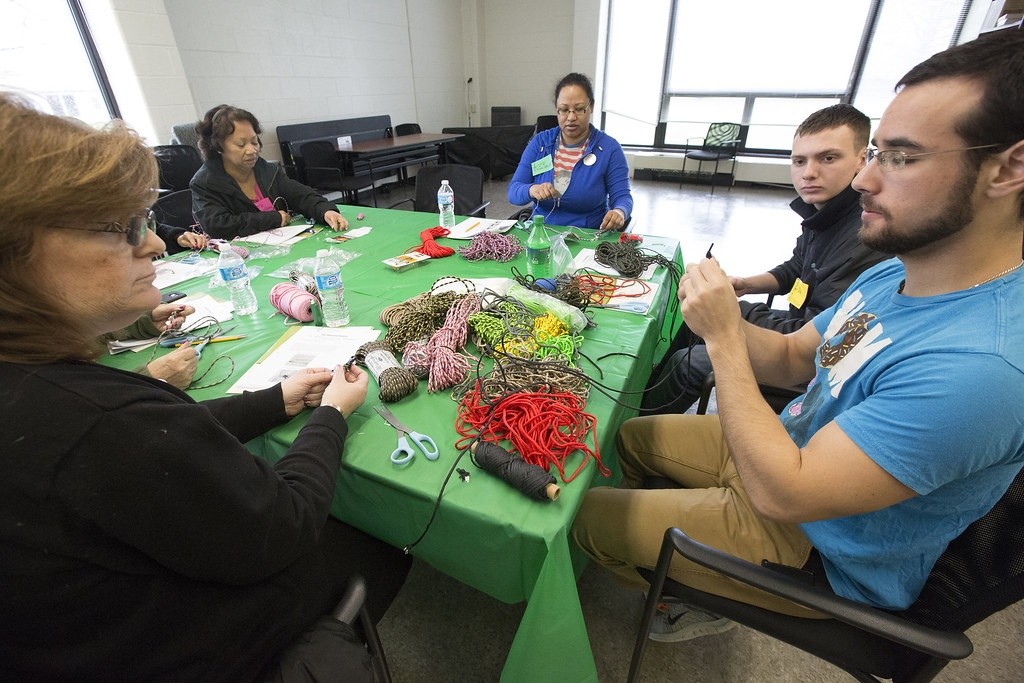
[174,334,246,347]
[305,229,315,233]
[465,221,481,233]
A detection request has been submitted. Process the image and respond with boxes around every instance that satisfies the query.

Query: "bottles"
[437,180,455,229]
[310,300,323,326]
[218,243,258,316]
[526,215,552,280]
[314,249,350,328]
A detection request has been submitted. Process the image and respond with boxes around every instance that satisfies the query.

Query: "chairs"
[537,115,560,135]
[412,165,487,216]
[298,141,378,207]
[152,186,205,251]
[492,106,523,128]
[601,290,1024,683]
[266,540,414,682]
[155,144,203,188]
[396,122,441,186]
[680,121,749,195]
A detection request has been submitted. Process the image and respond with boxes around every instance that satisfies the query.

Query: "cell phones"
[160,290,187,304]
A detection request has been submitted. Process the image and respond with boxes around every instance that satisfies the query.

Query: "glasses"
[19,208,157,247]
[866,140,1005,173]
[556,100,591,115]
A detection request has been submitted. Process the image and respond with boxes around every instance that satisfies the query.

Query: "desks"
[93,200,688,682]
[336,132,467,208]
[441,123,536,180]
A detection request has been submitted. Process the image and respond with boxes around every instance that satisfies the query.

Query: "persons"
[641,103,892,416]
[569,27,1024,641]
[186,102,352,240]
[0,87,411,683]
[509,72,633,230]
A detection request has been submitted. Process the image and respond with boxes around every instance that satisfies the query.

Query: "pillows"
[706,122,740,149]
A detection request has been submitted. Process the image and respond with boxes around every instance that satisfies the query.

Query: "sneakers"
[646,596,739,642]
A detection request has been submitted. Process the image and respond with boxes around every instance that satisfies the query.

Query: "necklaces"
[965,258,1024,290]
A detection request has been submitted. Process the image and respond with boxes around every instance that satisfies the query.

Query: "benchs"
[274,114,402,201]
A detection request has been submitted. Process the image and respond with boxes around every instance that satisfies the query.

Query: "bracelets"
[324,403,344,415]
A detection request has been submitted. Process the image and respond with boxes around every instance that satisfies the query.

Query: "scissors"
[182,251,205,266]
[160,324,249,360]
[512,220,535,238]
[375,403,441,464]
